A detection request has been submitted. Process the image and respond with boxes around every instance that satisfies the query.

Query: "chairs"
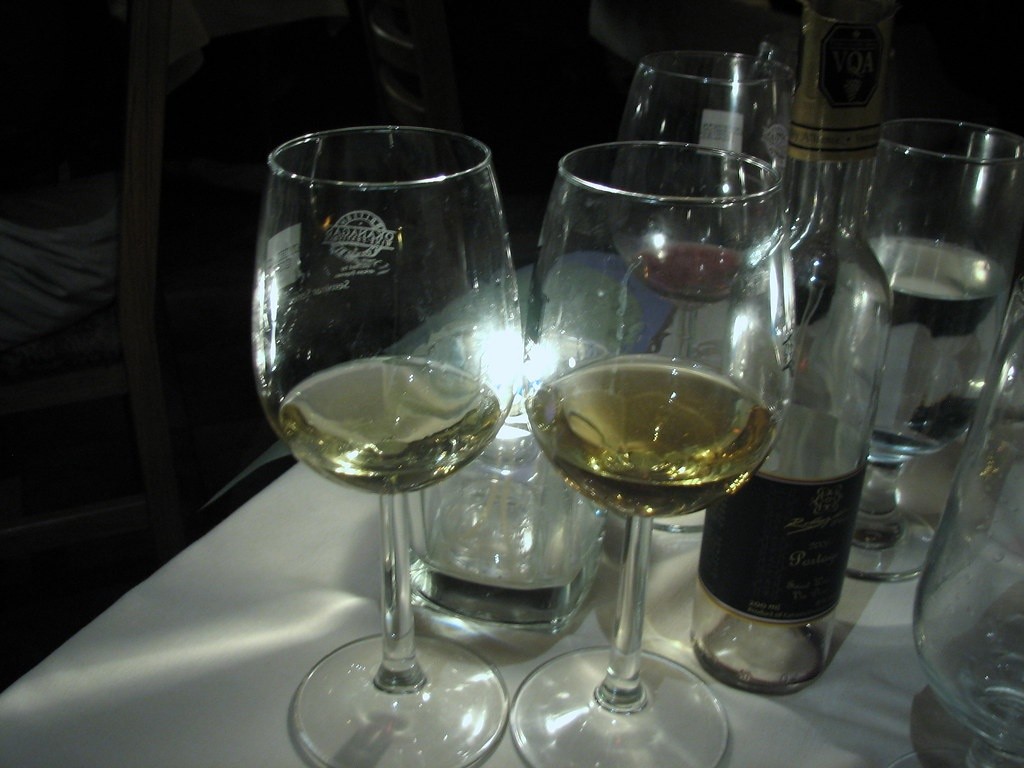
[359,0,617,267]
[116,0,402,572]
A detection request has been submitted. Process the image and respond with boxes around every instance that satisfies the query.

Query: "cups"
[406,327,607,632]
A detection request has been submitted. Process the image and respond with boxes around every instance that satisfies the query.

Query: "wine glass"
[609,50,793,534]
[252,126,523,768]
[887,268,1024,768]
[510,141,794,768]
[844,117,1024,581]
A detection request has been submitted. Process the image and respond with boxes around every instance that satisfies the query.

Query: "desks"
[0,250,1024,768]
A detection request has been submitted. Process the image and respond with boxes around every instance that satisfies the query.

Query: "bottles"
[690,0,891,692]
